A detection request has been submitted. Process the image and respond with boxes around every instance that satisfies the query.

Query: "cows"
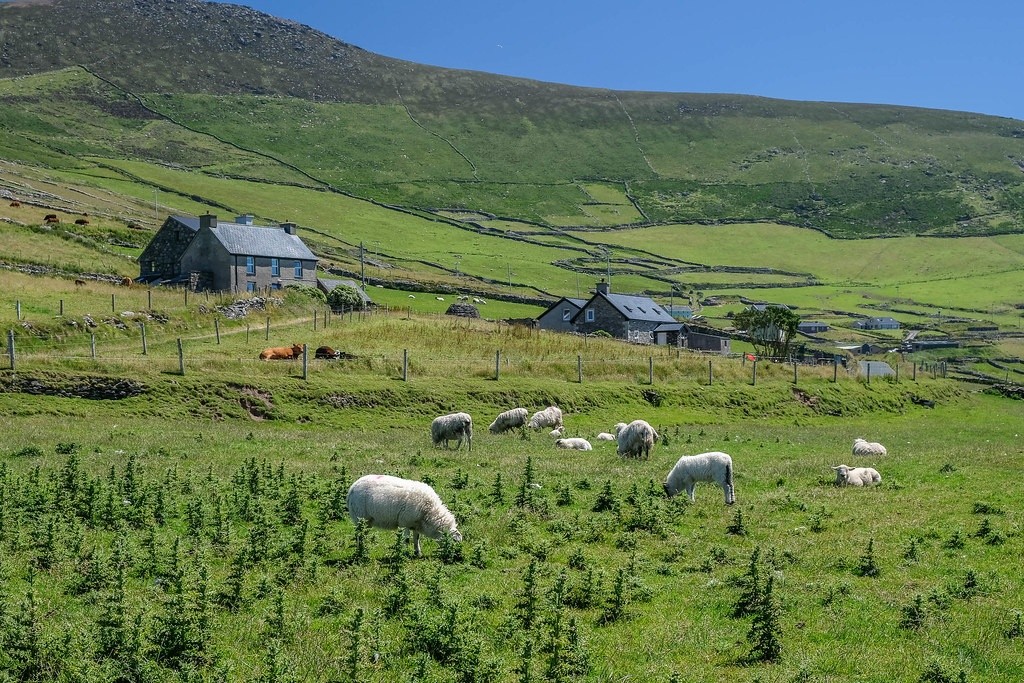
[9,200,133,288]
[315,345,352,360]
[260,342,303,361]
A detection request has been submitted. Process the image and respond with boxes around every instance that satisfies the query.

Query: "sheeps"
[489,407,529,435]
[832,464,881,487]
[376,283,487,306]
[597,420,659,460]
[529,406,593,452]
[663,452,737,506]
[431,412,473,452]
[852,438,889,456]
[346,473,463,560]
